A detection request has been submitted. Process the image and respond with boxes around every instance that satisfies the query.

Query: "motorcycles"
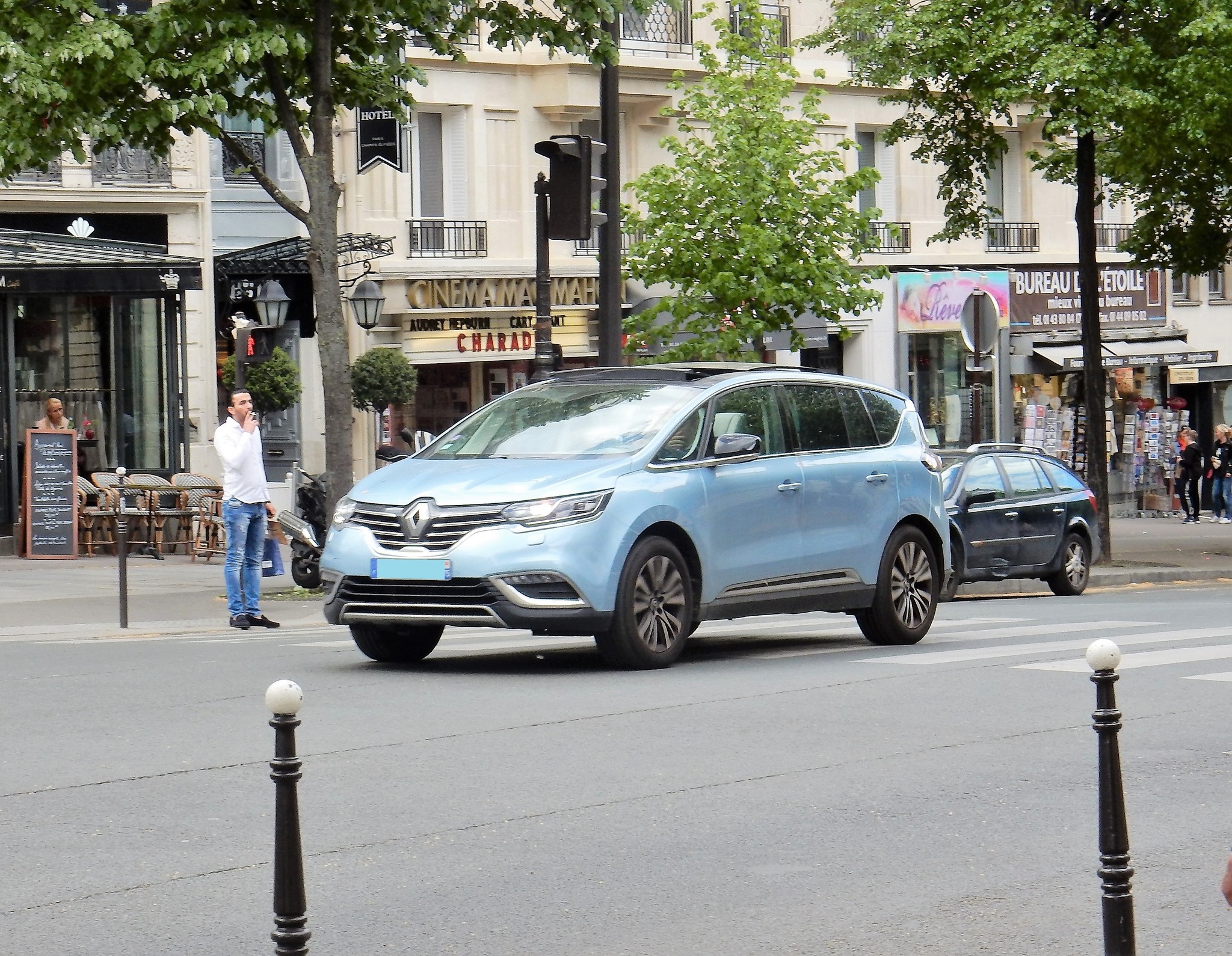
[283,428,464,588]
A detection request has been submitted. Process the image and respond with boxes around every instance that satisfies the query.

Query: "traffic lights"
[535,135,590,241]
[236,327,279,362]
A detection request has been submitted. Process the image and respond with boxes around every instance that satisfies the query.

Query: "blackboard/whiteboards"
[17,428,79,560]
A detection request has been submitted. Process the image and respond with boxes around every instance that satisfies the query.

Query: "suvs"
[316,366,951,669]
[924,440,1102,595]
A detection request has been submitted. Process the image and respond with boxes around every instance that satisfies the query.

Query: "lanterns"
[1168,396,1187,410]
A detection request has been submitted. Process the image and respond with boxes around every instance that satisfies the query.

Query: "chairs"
[128,473,191,555]
[75,475,116,557]
[713,412,748,437]
[170,472,224,554]
[91,472,151,554]
[190,491,226,563]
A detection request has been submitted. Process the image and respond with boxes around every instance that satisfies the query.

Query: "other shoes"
[245,614,280,629]
[230,615,250,628]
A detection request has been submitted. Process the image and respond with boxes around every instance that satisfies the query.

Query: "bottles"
[67,417,75,430]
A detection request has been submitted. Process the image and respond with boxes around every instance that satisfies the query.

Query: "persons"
[1209,424,1232,524]
[214,388,281,628]
[666,410,700,455]
[1176,430,1202,524]
[35,397,87,480]
[1178,425,1192,459]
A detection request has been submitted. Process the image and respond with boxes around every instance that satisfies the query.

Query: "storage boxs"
[1137,494,1171,511]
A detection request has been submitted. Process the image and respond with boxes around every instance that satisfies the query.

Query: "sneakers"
[1209,515,1221,523]
[1182,516,1194,524]
[1193,517,1200,524]
[1219,518,1231,523]
